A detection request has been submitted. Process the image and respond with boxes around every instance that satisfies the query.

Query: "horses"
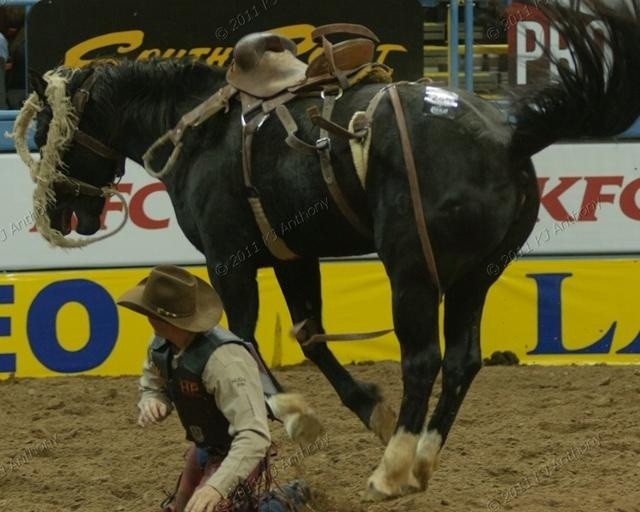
[26,0,640,505]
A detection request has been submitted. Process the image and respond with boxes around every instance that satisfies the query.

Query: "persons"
[114,263,314,512]
[4,23,15,108]
[0,32,9,107]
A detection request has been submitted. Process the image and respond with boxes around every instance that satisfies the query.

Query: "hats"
[117,263,223,334]
